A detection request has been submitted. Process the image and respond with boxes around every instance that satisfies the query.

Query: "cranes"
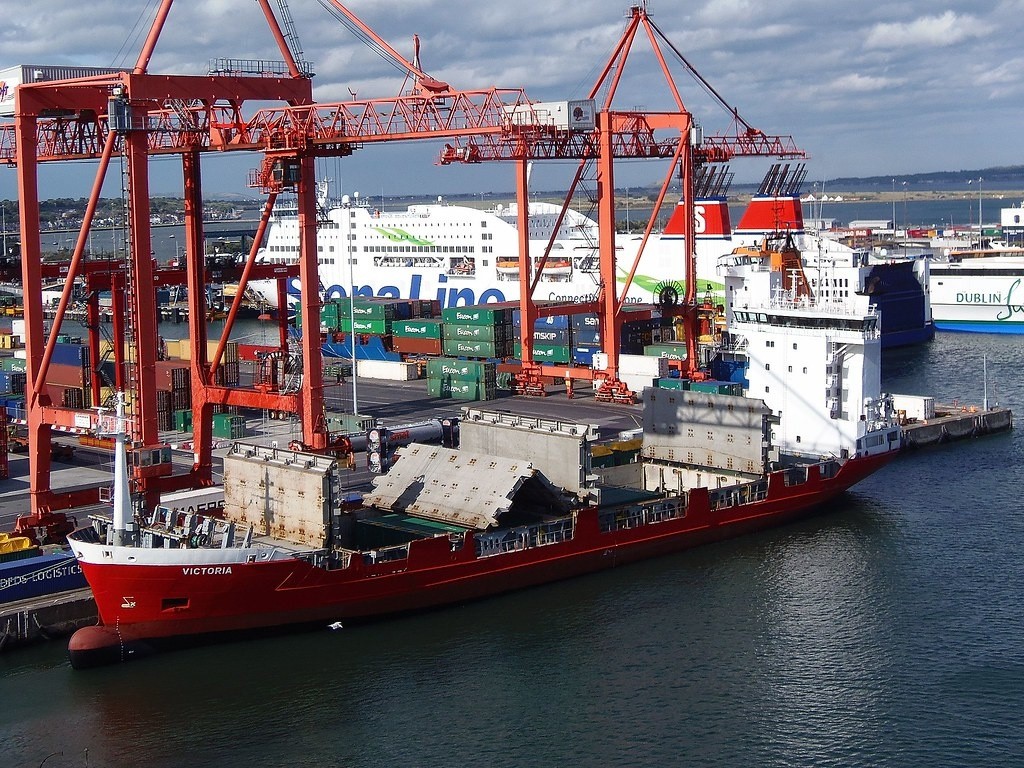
[431,0,810,410]
[0,2,565,548]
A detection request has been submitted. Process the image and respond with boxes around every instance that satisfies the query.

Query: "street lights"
[889,178,897,254]
[966,180,973,252]
[978,176,986,250]
[902,181,909,259]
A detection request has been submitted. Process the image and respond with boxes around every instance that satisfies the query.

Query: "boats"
[1,185,1024,657]
